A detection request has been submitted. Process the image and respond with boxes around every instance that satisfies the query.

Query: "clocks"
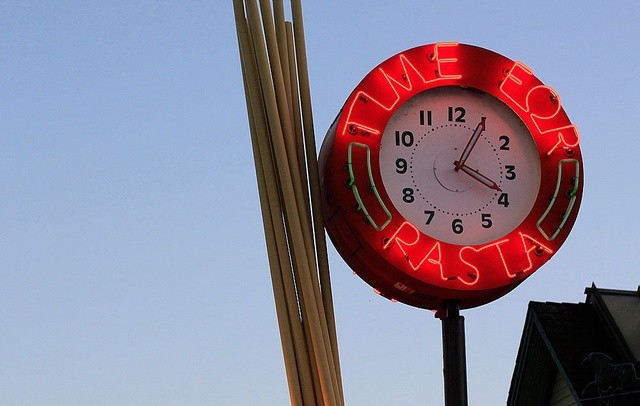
[317,41,584,310]
